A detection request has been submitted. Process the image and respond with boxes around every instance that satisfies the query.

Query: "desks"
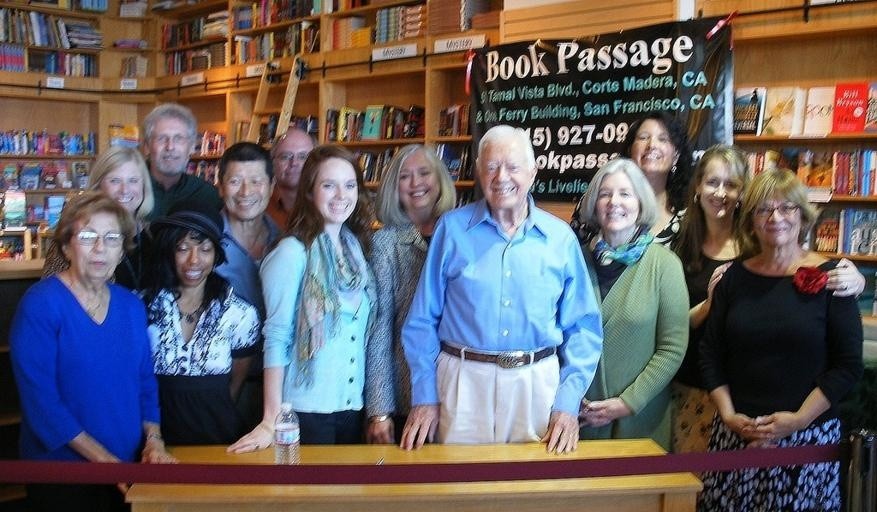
[122,437,706,512]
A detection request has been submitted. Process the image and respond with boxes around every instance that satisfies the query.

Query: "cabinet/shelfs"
[102,1,155,153]
[321,0,425,231]
[155,0,230,163]
[230,0,321,165]
[0,0,102,240]
[425,1,502,190]
[700,14,876,266]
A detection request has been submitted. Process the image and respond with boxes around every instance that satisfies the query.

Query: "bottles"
[0,128,94,154]
[273,402,301,465]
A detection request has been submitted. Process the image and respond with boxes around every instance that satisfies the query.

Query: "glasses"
[76,231,126,246]
[752,204,799,218]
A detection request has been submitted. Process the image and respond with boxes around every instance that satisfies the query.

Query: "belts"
[440,343,555,370]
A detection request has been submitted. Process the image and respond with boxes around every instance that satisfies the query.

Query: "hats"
[150,201,225,246]
